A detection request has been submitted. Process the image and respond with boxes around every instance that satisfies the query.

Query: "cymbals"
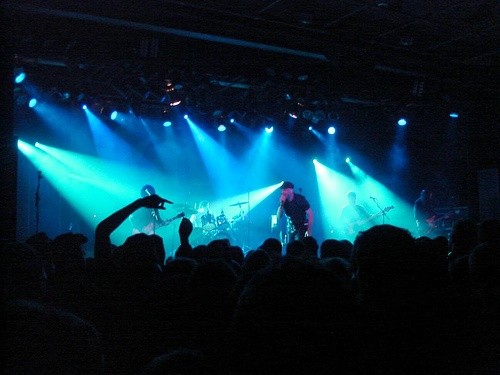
[177,207,199,215]
[228,201,249,207]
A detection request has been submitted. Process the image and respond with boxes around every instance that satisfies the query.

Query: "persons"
[0,194,282,375]
[340,192,376,244]
[282,219,500,375]
[277,181,313,242]
[129,185,166,235]
[414,189,439,239]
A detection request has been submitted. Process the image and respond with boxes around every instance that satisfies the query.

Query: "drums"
[215,215,230,230]
[201,214,216,231]
[189,227,204,243]
[214,231,233,245]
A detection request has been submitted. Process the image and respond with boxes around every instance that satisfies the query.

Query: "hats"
[281,182,294,189]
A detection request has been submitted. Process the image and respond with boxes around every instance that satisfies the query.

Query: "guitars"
[132,211,186,236]
[417,210,461,235]
[341,205,394,238]
